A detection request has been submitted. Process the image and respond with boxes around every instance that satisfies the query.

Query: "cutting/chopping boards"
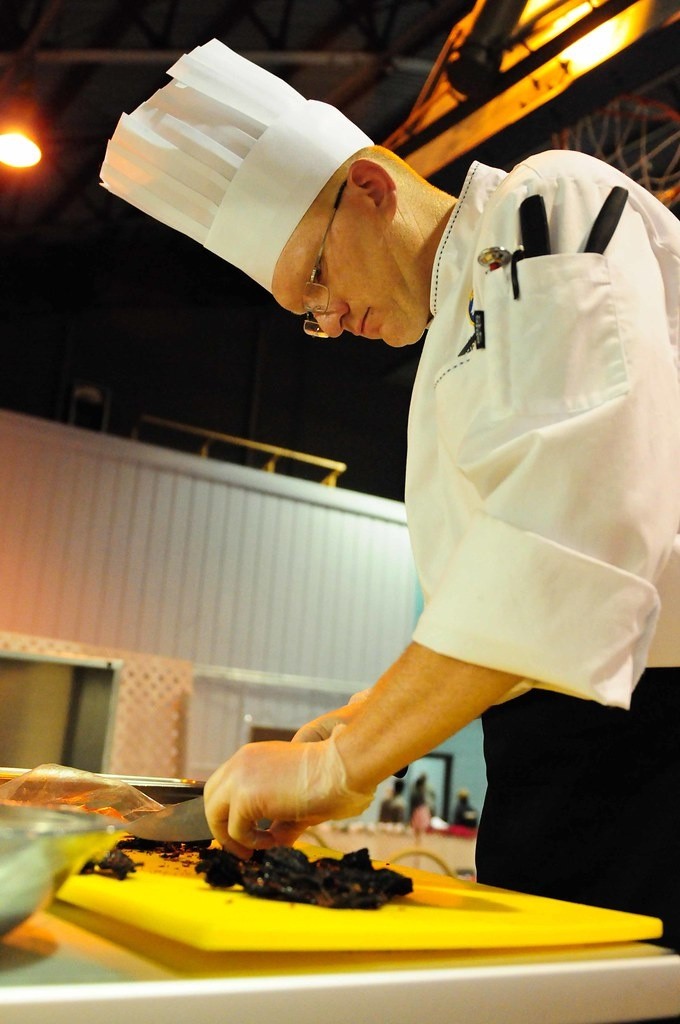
[56,828,664,951]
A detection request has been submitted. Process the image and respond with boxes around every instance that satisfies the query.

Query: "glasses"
[297,168,350,342]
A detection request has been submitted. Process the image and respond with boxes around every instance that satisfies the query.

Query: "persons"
[99,37,680,955]
[359,770,480,843]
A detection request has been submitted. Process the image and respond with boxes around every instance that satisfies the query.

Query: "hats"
[98,36,376,295]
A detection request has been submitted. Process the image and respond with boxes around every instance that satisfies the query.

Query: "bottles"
[454,791,479,829]
[381,778,407,823]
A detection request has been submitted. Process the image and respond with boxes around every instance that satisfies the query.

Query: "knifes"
[126,796,214,842]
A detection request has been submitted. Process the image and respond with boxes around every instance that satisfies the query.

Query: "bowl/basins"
[0,802,128,935]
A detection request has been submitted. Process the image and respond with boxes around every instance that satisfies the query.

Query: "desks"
[0,812,680,1024]
